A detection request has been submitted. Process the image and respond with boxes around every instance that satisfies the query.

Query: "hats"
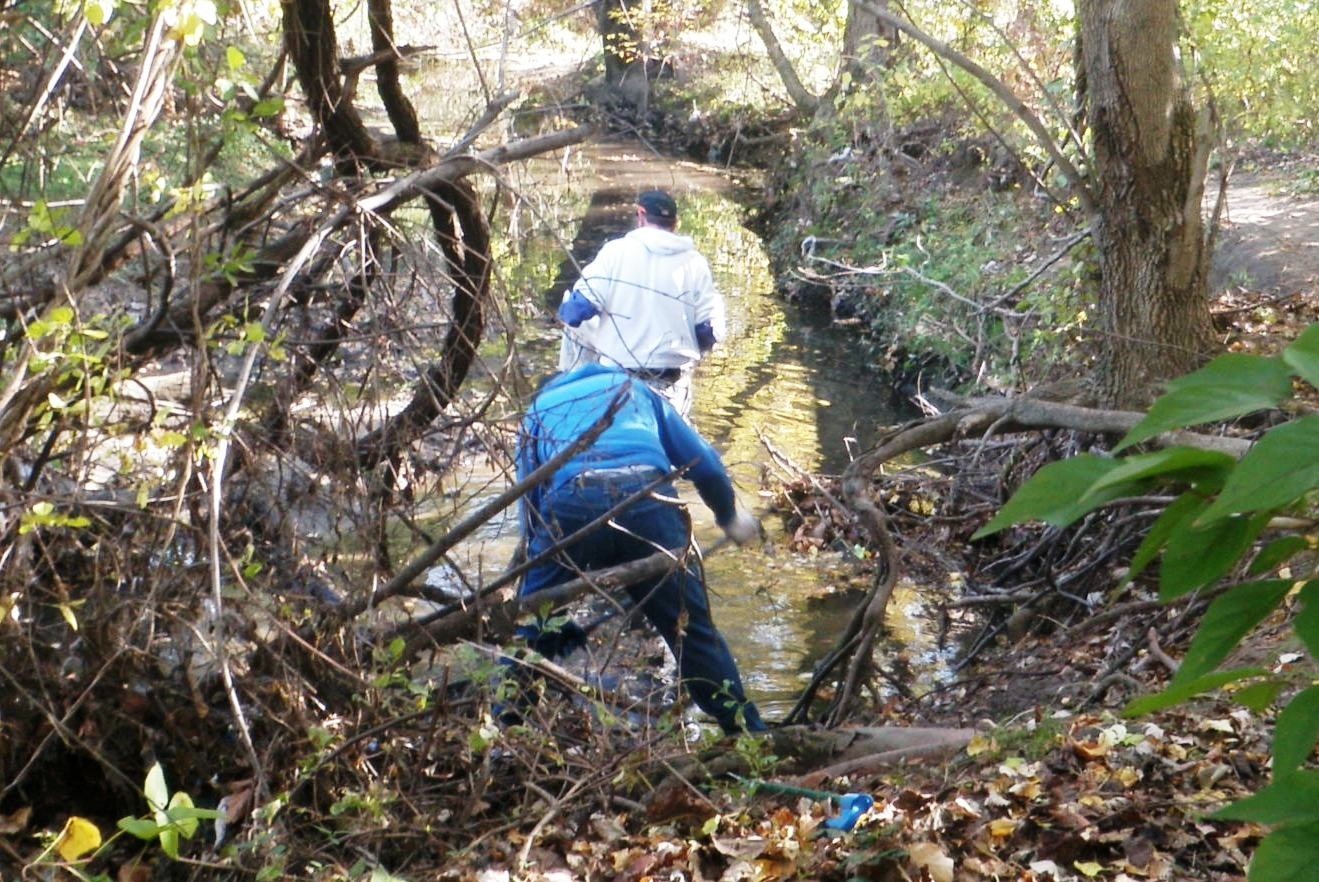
[636,190,676,220]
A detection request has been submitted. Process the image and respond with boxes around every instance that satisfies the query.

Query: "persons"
[493,362,767,737]
[558,191,727,487]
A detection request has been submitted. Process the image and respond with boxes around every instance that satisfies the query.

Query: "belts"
[567,468,664,487]
